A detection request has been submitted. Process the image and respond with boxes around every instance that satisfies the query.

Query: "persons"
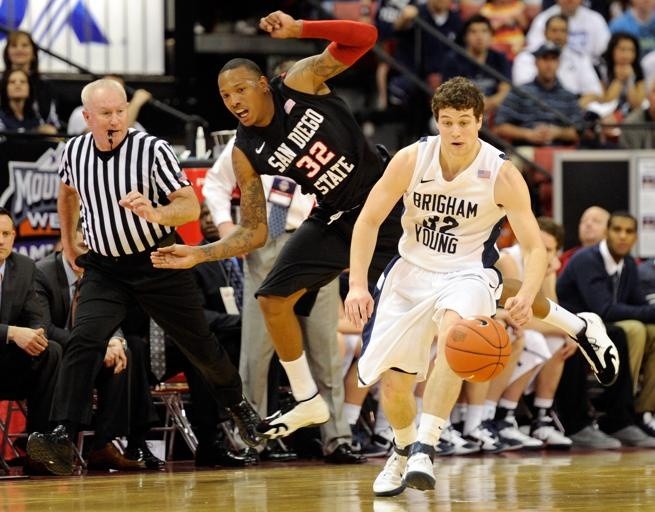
[0,0,655,494]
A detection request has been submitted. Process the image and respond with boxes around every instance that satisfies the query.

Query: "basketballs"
[445,316,512,383]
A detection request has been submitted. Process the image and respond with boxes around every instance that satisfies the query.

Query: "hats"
[534,43,562,57]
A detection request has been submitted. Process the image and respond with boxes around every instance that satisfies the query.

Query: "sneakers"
[436,411,654,455]
[373,435,436,497]
[567,312,620,387]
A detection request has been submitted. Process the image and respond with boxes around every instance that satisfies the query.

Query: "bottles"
[195,126,206,160]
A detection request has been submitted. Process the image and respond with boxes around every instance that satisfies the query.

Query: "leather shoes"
[22,423,166,477]
[195,387,387,464]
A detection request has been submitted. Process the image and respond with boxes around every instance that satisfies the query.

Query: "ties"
[226,259,244,311]
[268,203,287,239]
[72,278,83,330]
[150,317,166,382]
